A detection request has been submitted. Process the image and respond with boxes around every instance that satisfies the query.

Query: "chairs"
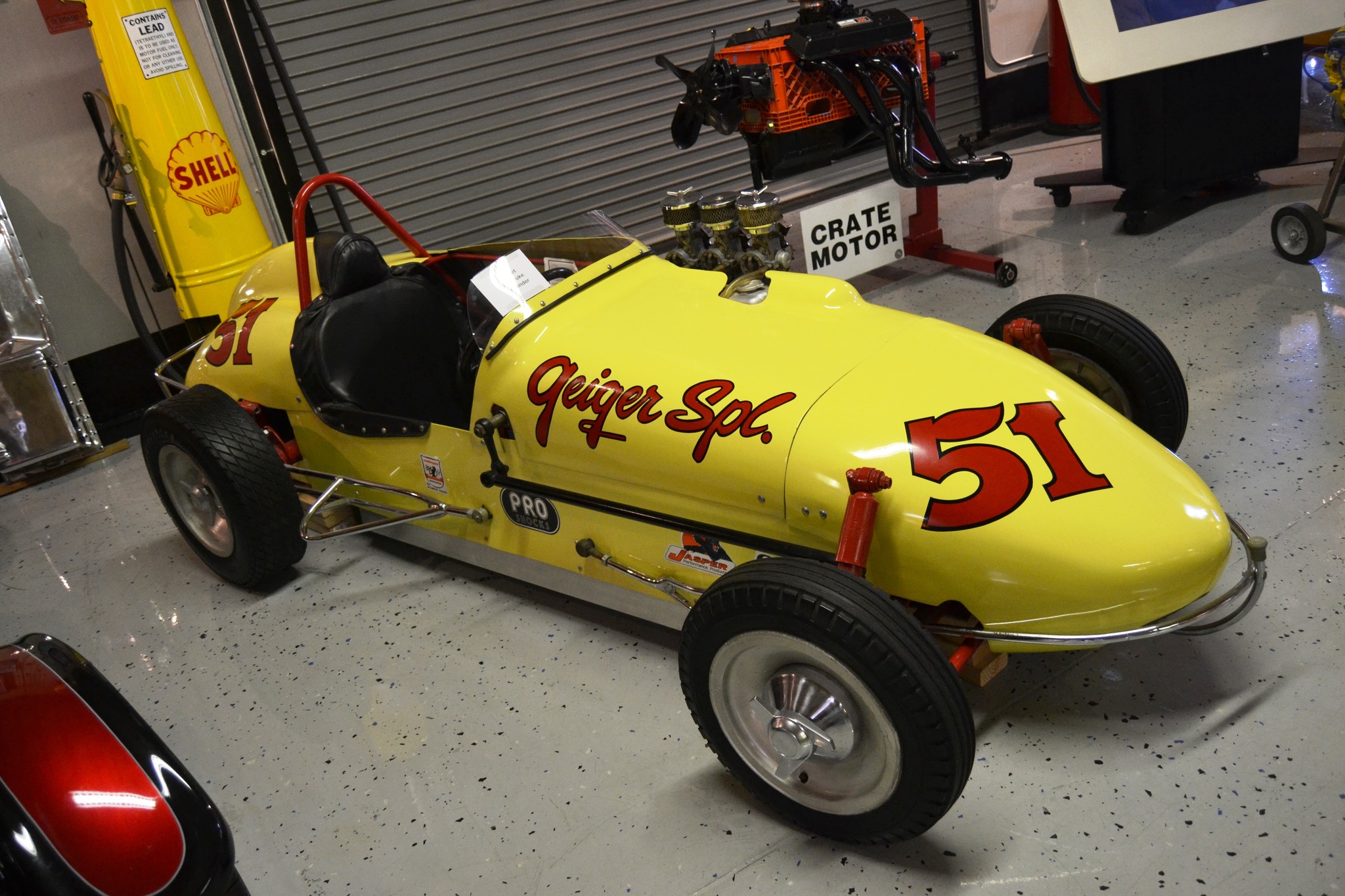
[295,232,472,428]
